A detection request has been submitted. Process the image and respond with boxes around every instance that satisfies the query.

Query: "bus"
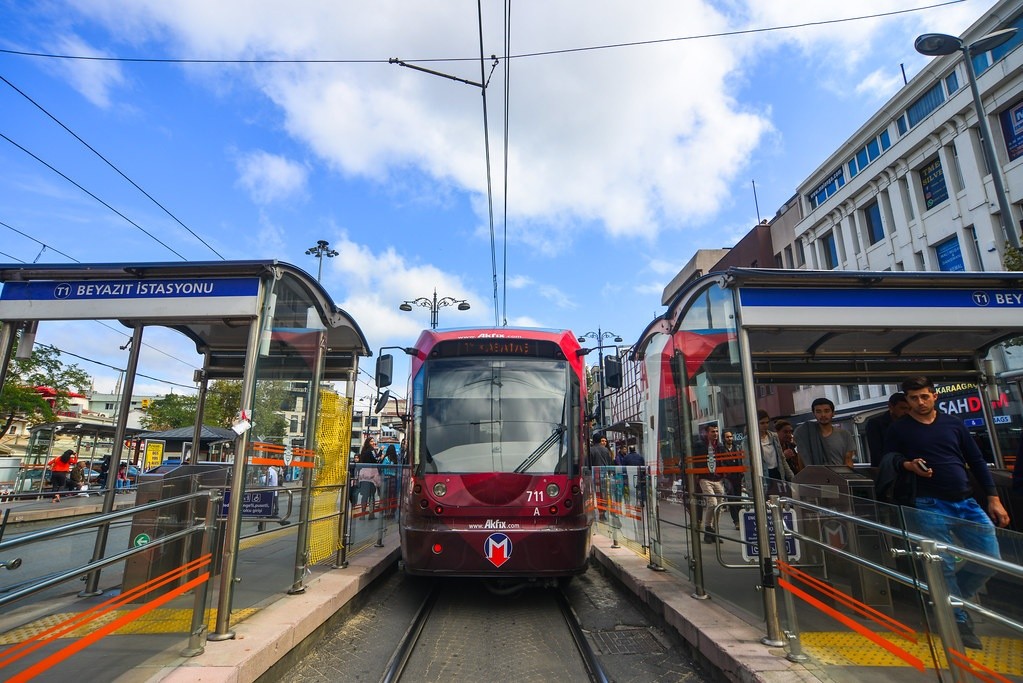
[375,325,622,593]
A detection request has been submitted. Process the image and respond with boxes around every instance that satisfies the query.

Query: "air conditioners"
[98,412,109,418]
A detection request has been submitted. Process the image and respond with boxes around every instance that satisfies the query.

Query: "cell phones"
[917,460,928,473]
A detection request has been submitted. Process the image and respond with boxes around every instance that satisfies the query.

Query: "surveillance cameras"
[231,420,251,435]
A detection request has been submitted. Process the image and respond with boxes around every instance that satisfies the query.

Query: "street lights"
[577,323,623,438]
[304,240,340,281]
[399,286,471,330]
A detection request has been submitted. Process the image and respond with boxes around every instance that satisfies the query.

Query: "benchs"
[30,475,136,492]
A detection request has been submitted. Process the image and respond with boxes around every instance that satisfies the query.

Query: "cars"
[16,465,140,491]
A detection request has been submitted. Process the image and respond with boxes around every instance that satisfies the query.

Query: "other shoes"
[97,491,106,497]
[704,539,713,544]
[368,516,377,521]
[712,536,724,543]
[599,513,608,523]
[359,517,365,521]
[52,494,60,503]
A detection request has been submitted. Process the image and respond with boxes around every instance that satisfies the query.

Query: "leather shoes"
[958,623,982,650]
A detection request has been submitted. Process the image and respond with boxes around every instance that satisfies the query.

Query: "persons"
[866,394,928,599]
[693,397,854,576]
[47,449,132,504]
[585,434,646,527]
[883,377,1010,648]
[181,458,190,465]
[350,437,401,521]
[260,466,284,515]
